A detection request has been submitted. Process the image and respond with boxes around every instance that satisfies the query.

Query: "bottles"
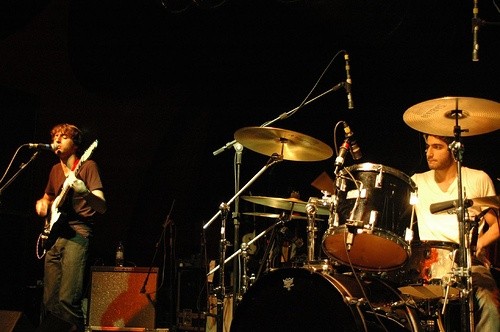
[116,241,124,267]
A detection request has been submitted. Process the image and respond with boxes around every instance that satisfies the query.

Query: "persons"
[36,124,106,332]
[398,133,500,332]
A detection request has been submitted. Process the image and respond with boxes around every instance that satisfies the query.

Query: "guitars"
[41,138,100,252]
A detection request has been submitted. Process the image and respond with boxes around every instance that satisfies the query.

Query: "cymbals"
[241,195,330,216]
[234,126,334,162]
[471,194,500,210]
[242,210,325,223]
[401,96,500,137]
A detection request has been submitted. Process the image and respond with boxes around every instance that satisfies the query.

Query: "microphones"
[473,0,478,62]
[344,52,354,109]
[140,287,146,293]
[24,143,57,152]
[464,208,491,234]
[343,122,362,160]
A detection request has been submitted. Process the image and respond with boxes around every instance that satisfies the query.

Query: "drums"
[229,267,421,332]
[321,162,419,271]
[383,239,467,302]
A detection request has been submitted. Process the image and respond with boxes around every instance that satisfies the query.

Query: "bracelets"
[82,190,91,196]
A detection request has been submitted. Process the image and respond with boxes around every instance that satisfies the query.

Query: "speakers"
[85,266,171,330]
[0,311,34,332]
